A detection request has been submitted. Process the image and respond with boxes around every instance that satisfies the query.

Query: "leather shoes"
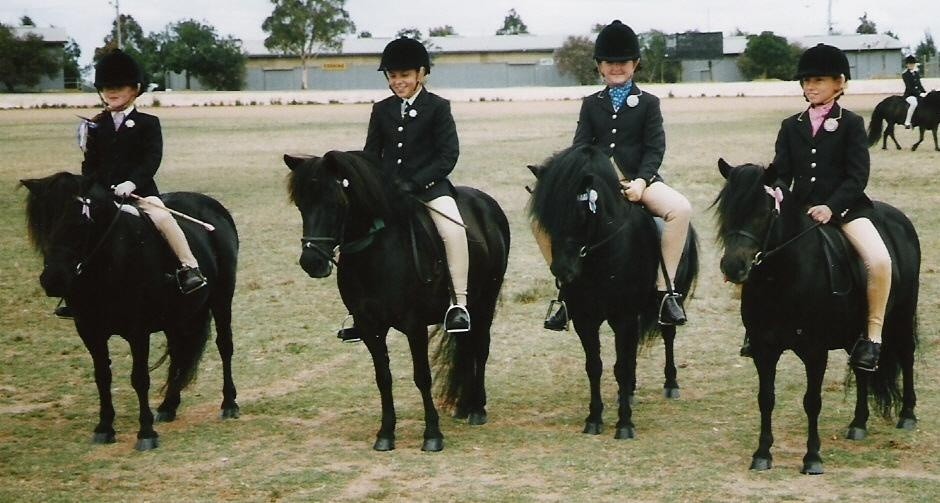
[741,334,751,358]
[544,306,570,329]
[905,125,910,129]
[657,291,686,324]
[446,307,470,330]
[338,328,360,340]
[55,307,73,317]
[177,266,205,295]
[852,342,880,370]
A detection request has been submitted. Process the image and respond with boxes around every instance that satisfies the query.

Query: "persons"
[337,36,470,340]
[741,43,892,372]
[544,20,691,331]
[901,55,928,129]
[55,48,204,318]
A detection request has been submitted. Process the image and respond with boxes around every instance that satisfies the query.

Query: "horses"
[709,157,921,474]
[15,171,239,450]
[867,90,940,150]
[523,140,701,439]
[283,149,511,451]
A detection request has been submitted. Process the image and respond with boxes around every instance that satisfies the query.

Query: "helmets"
[377,37,430,72]
[94,52,140,85]
[594,20,640,62]
[905,55,917,63]
[794,43,850,82]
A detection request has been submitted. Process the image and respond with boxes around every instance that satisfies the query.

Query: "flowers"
[409,108,417,117]
[125,119,136,128]
[823,118,838,132]
[626,94,640,108]
